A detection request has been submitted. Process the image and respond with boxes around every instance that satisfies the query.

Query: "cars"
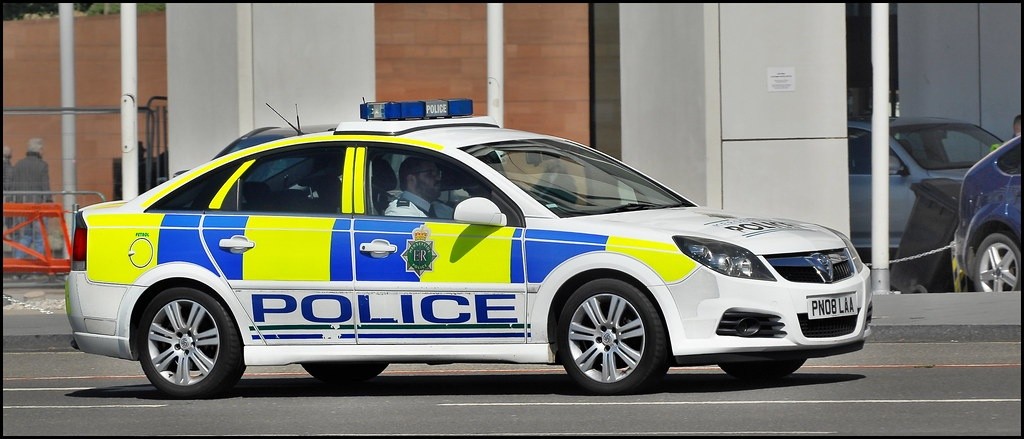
[848,113,1005,292]
[63,94,874,397]
[953,134,1021,292]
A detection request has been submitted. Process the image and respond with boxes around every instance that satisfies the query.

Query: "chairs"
[372,159,398,214]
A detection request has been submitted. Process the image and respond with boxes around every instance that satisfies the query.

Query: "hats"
[26,138,43,152]
[3,146,12,158]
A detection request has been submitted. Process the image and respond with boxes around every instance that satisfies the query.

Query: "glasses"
[412,170,442,178]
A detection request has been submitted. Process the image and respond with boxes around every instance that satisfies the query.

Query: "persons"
[384,158,456,221]
[4,136,52,279]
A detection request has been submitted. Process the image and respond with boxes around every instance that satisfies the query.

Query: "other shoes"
[12,272,26,281]
[37,273,48,279]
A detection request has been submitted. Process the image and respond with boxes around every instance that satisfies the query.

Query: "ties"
[430,204,437,219]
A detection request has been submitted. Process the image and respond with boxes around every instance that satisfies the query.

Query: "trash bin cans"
[891,175,961,293]
